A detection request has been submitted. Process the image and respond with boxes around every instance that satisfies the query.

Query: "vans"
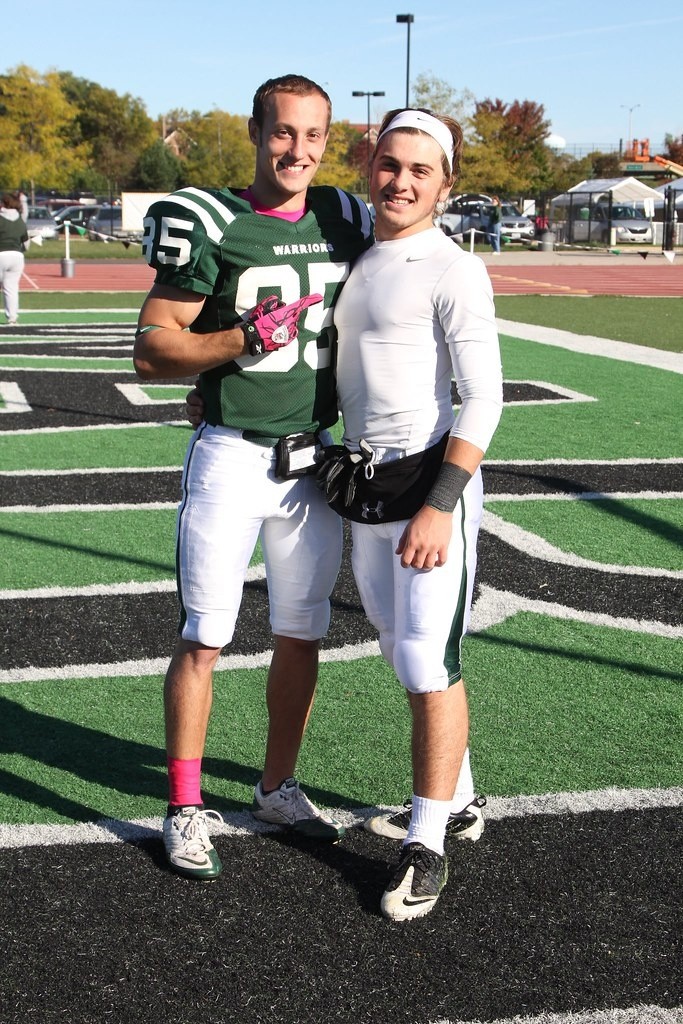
[48,200,83,216]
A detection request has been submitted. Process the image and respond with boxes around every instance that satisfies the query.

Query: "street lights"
[396,14,414,109]
[352,91,385,203]
[620,104,641,162]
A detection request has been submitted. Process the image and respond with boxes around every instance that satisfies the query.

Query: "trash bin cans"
[539,230,555,252]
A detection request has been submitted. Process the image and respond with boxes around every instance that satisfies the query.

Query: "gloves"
[314,438,375,507]
[239,292,324,356]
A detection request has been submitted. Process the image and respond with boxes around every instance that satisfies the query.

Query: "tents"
[550,177,669,248]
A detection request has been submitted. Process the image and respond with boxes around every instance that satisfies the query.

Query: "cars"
[88,205,122,242]
[25,206,59,240]
[591,200,653,245]
[433,193,499,236]
[462,203,536,245]
[51,205,97,234]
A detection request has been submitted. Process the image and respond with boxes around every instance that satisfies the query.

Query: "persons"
[488,195,502,256]
[132,73,375,880]
[0,193,30,324]
[327,108,503,921]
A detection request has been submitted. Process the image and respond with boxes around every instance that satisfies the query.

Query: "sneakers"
[364,796,486,842]
[382,842,449,921]
[162,805,224,878]
[251,777,345,839]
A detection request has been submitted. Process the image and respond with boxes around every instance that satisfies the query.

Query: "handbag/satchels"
[328,427,453,524]
